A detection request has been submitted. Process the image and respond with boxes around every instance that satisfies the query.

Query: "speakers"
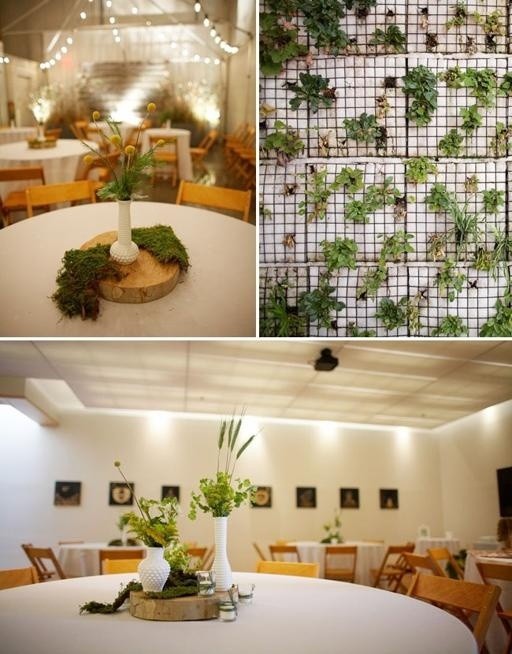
[497,467,512,517]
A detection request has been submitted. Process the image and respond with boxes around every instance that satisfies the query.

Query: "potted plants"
[187,403,265,592]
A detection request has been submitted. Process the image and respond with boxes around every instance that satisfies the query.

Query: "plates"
[112,487,130,504]
[254,490,269,506]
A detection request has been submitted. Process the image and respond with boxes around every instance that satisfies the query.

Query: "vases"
[110,200,139,266]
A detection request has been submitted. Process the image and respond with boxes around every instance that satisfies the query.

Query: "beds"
[0,201,256,336]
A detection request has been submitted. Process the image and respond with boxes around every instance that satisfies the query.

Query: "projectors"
[314,357,338,370]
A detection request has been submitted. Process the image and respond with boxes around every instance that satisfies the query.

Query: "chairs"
[0,538,512,654]
[0,122,256,225]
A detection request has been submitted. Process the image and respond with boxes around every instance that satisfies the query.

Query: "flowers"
[113,460,180,547]
[80,103,165,203]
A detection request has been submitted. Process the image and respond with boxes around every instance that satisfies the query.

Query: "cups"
[236,580,255,604]
[215,583,237,621]
[444,529,453,540]
[330,537,338,546]
[195,570,217,599]
